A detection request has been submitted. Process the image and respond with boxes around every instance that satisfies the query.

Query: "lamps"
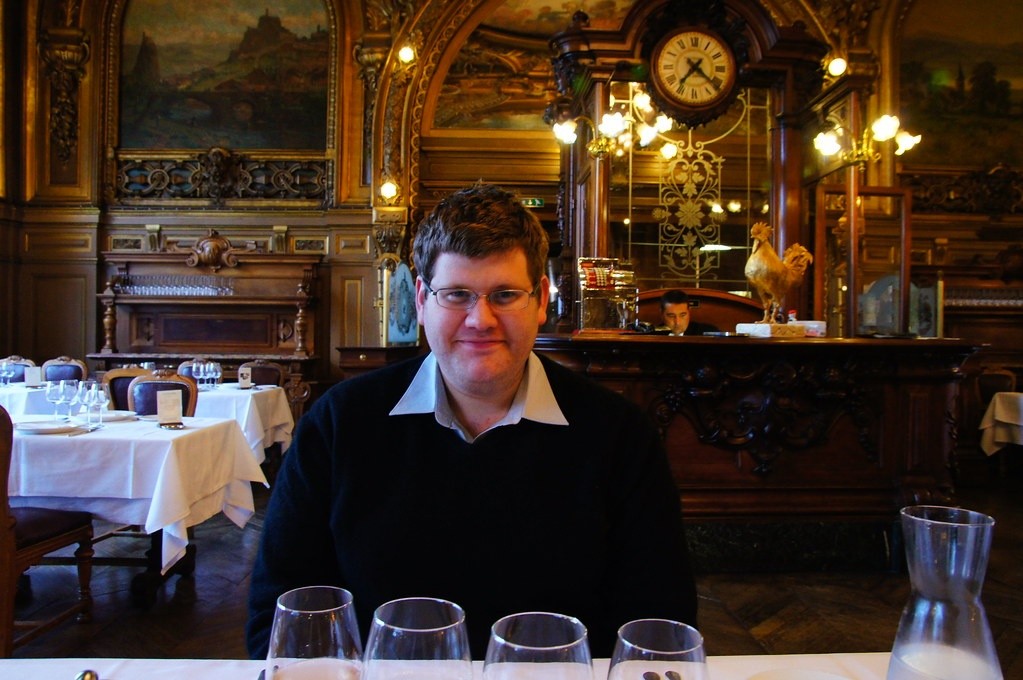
[812,115,922,173]
[553,111,627,158]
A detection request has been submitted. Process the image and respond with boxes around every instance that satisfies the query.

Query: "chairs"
[0,356,286,659]
[0,405,93,657]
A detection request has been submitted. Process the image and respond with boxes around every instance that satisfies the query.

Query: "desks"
[90,381,294,491]
[8,415,270,610]
[0,381,78,415]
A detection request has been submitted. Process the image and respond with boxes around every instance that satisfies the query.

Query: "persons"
[651,289,721,336]
[246,179,700,661]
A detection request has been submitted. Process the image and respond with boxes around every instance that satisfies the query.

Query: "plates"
[77,411,137,422]
[15,422,78,434]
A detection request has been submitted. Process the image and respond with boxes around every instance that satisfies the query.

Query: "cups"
[265,586,364,680]
[607,619,710,680]
[123,362,155,370]
[157,390,182,428]
[24,367,40,388]
[482,612,596,680]
[364,597,472,680]
[238,367,251,389]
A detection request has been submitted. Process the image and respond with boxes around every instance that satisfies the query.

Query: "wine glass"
[130,276,234,297]
[0,362,15,389]
[193,362,221,391]
[47,380,111,432]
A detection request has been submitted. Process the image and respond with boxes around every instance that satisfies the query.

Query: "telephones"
[620,322,673,336]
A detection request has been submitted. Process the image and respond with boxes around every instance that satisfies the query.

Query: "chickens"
[744,221,813,324]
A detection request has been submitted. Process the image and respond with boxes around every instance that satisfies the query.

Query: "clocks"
[640,0,751,131]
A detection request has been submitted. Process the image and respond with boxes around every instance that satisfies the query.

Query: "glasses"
[423,279,541,311]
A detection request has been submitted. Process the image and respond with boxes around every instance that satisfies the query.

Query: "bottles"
[163,365,177,376]
[581,262,609,268]
[886,505,1004,680]
